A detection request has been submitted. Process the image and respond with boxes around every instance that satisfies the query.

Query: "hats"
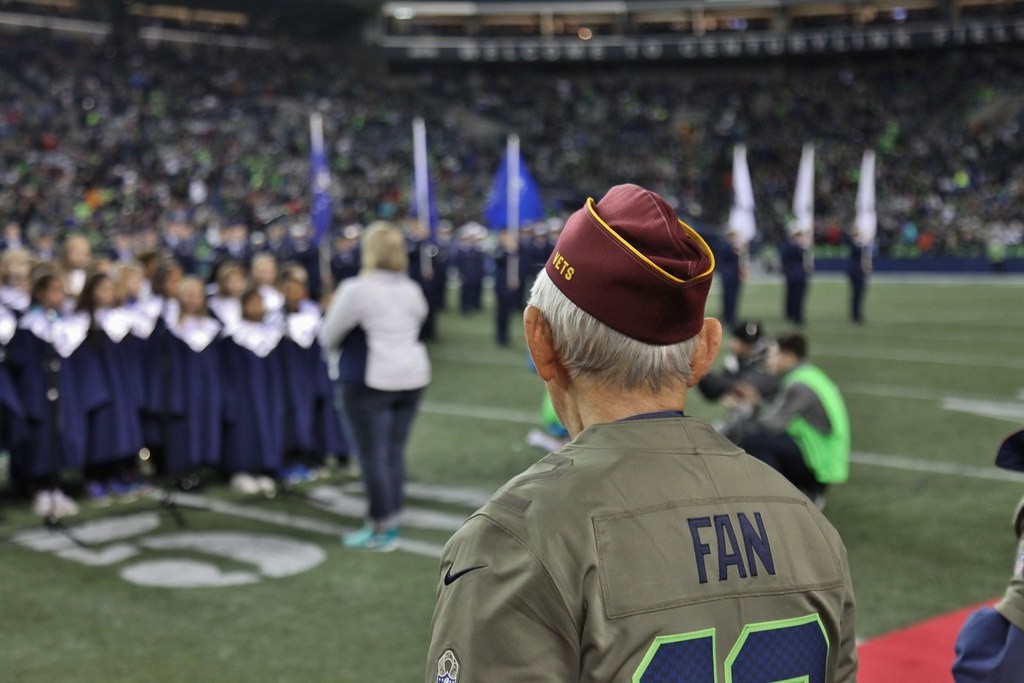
[545,183,716,346]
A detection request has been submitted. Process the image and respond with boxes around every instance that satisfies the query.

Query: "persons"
[0,0,1024,516]
[315,222,433,552]
[426,185,859,683]
[952,428,1024,683]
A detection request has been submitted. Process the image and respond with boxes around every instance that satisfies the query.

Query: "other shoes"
[344,527,401,553]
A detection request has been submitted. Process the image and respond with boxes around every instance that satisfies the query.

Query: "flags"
[308,147,330,247]
[727,150,757,243]
[852,151,877,245]
[791,146,815,249]
[410,150,438,245]
[482,151,544,229]
